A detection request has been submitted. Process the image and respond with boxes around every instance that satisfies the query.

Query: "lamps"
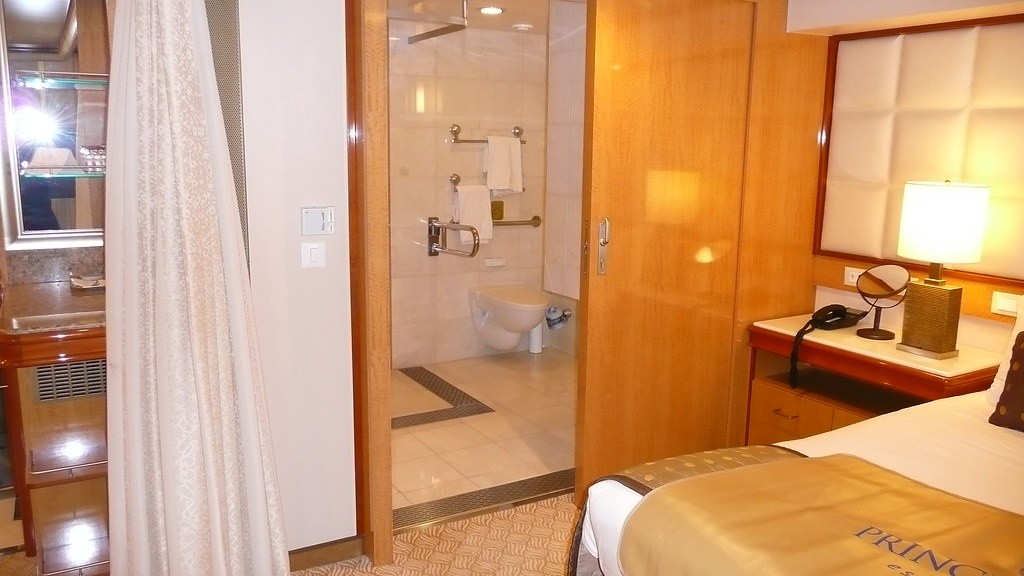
[896,179,991,361]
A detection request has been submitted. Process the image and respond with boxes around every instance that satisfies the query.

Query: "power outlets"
[844,266,866,287]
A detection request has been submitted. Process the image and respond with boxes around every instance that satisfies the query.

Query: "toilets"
[468,284,549,350]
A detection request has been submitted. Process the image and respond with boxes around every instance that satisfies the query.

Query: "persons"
[11,85,64,231]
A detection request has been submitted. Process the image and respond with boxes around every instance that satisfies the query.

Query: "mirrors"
[0,0,117,251]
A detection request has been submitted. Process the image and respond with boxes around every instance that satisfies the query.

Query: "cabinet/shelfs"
[24,429,109,576]
[748,370,928,445]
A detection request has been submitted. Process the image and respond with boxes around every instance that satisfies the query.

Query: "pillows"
[985,294,1024,407]
[987,331,1024,433]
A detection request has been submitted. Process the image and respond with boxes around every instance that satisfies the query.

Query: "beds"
[562,389,1024,576]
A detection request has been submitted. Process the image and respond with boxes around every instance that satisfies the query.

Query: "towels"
[452,185,493,245]
[481,136,523,197]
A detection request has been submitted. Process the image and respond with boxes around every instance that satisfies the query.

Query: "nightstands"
[744,313,1003,446]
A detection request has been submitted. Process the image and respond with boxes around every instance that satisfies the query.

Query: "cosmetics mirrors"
[857,264,911,340]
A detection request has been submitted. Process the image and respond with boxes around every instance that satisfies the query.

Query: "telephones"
[810,304,869,330]
